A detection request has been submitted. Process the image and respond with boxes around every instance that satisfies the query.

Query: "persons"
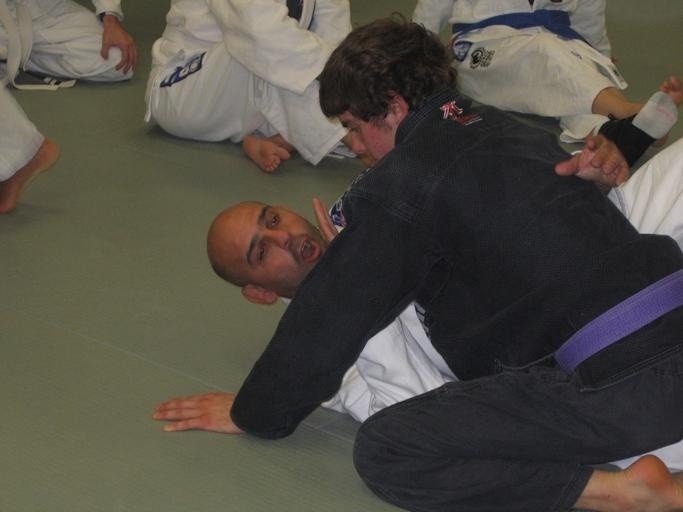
[0,0,139,85]
[204,135,683,477]
[145,0,356,173]
[149,18,683,512]
[0,77,58,216]
[412,0,683,148]
